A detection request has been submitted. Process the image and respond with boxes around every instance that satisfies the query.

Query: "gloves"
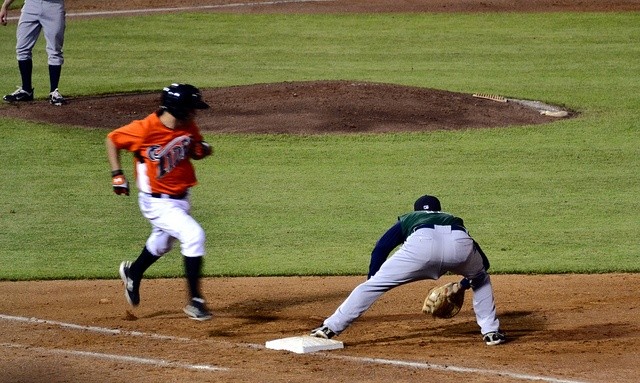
[111,170,130,197]
[199,142,211,158]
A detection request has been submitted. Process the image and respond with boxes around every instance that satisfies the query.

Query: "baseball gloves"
[422,282,465,319]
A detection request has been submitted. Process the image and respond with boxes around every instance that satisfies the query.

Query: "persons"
[312,195,506,347]
[0,0,67,105]
[104,82,215,321]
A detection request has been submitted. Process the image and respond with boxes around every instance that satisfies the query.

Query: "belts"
[413,224,467,233]
[148,193,187,200]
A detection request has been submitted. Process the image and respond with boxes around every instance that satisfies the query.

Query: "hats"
[414,195,441,211]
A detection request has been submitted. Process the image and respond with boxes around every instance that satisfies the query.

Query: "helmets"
[159,83,209,122]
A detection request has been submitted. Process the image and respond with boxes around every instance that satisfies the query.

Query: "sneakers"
[118,260,142,306]
[2,85,34,102]
[310,323,334,340]
[49,87,66,106]
[182,297,212,321]
[483,330,506,345]
